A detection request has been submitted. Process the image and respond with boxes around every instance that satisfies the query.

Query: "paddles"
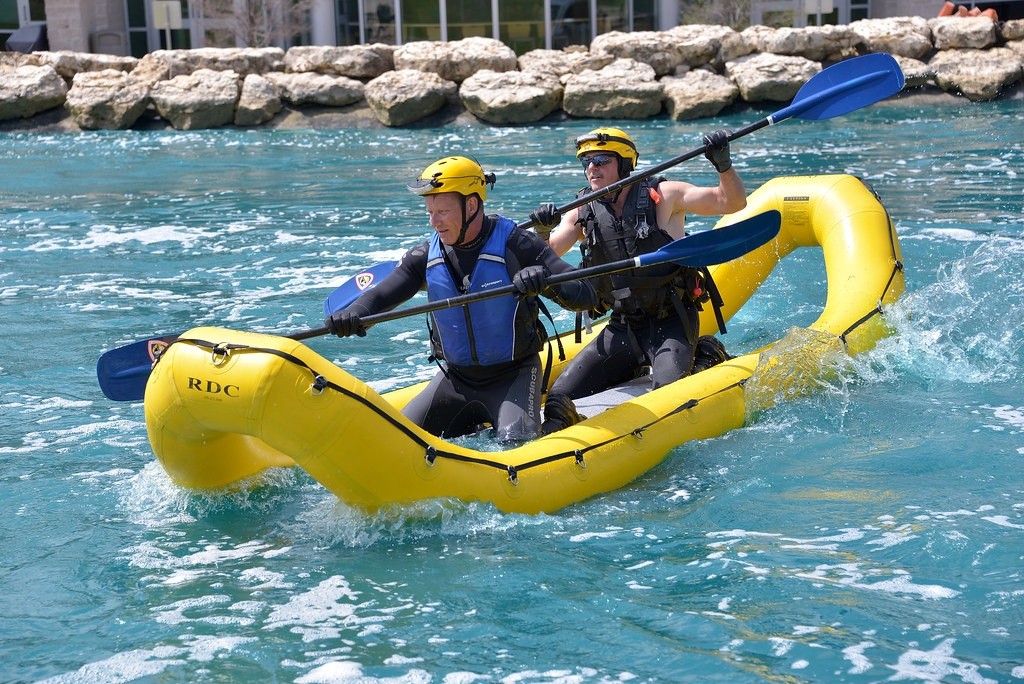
[324,53,905,332]
[94,209,783,402]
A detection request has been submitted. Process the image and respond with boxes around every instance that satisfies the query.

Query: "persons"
[323,154,597,441]
[529,128,746,398]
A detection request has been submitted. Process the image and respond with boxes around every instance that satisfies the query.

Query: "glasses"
[582,154,618,167]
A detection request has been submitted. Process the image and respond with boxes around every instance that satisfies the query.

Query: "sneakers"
[693,335,729,374]
[544,393,587,426]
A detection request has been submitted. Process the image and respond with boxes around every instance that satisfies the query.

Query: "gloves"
[529,203,561,235]
[512,266,561,299]
[702,129,733,172]
[325,310,366,338]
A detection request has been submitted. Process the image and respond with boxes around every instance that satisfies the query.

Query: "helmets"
[417,156,486,203]
[575,127,636,171]
